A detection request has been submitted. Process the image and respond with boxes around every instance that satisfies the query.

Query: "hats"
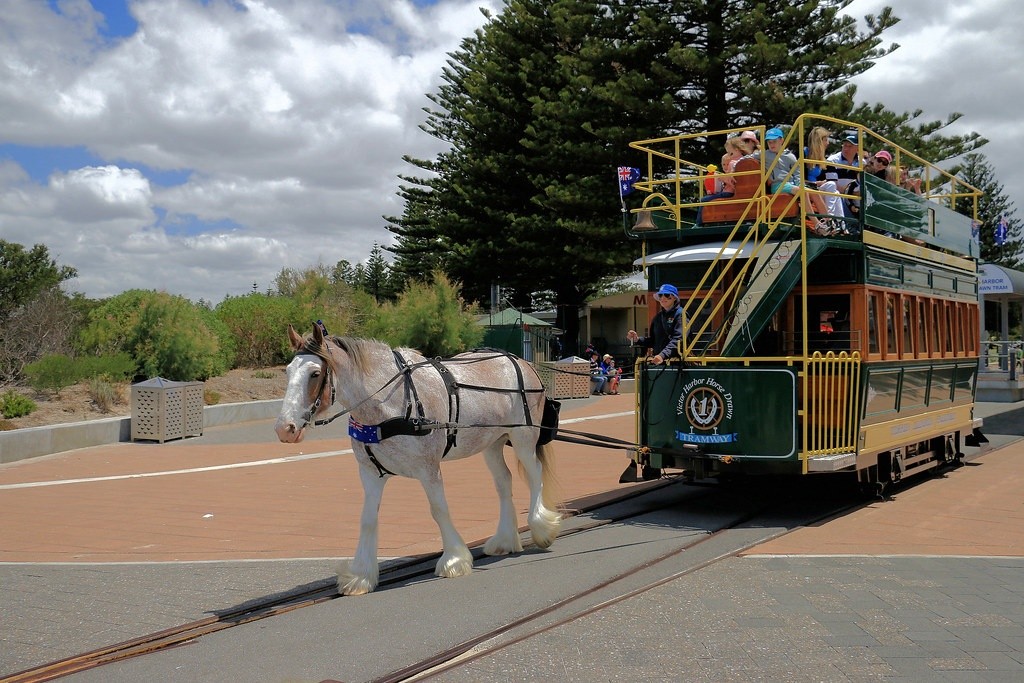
[588,344,594,348]
[592,351,600,356]
[764,128,783,143]
[653,284,680,304]
[875,151,891,164]
[740,131,759,145]
[603,354,613,362]
[842,135,859,145]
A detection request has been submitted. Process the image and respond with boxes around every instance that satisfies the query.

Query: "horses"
[275,321,564,597]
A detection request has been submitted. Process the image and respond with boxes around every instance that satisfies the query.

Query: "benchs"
[702,159,798,223]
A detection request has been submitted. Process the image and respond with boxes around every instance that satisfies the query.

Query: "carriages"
[273,114,992,595]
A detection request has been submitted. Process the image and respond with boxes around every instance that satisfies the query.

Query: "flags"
[994,216,1007,245]
[972,219,980,238]
[618,166,640,196]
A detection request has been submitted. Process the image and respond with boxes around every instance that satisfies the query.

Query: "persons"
[588,351,620,396]
[551,337,562,361]
[1016,345,1023,368]
[692,127,923,236]
[627,284,690,366]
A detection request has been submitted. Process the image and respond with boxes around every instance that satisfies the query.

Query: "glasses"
[744,139,750,143]
[657,294,671,299]
[864,157,869,159]
[876,158,888,166]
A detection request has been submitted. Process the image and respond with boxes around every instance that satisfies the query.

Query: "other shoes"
[815,219,841,236]
[691,224,702,228]
[609,392,620,395]
[592,391,606,395]
